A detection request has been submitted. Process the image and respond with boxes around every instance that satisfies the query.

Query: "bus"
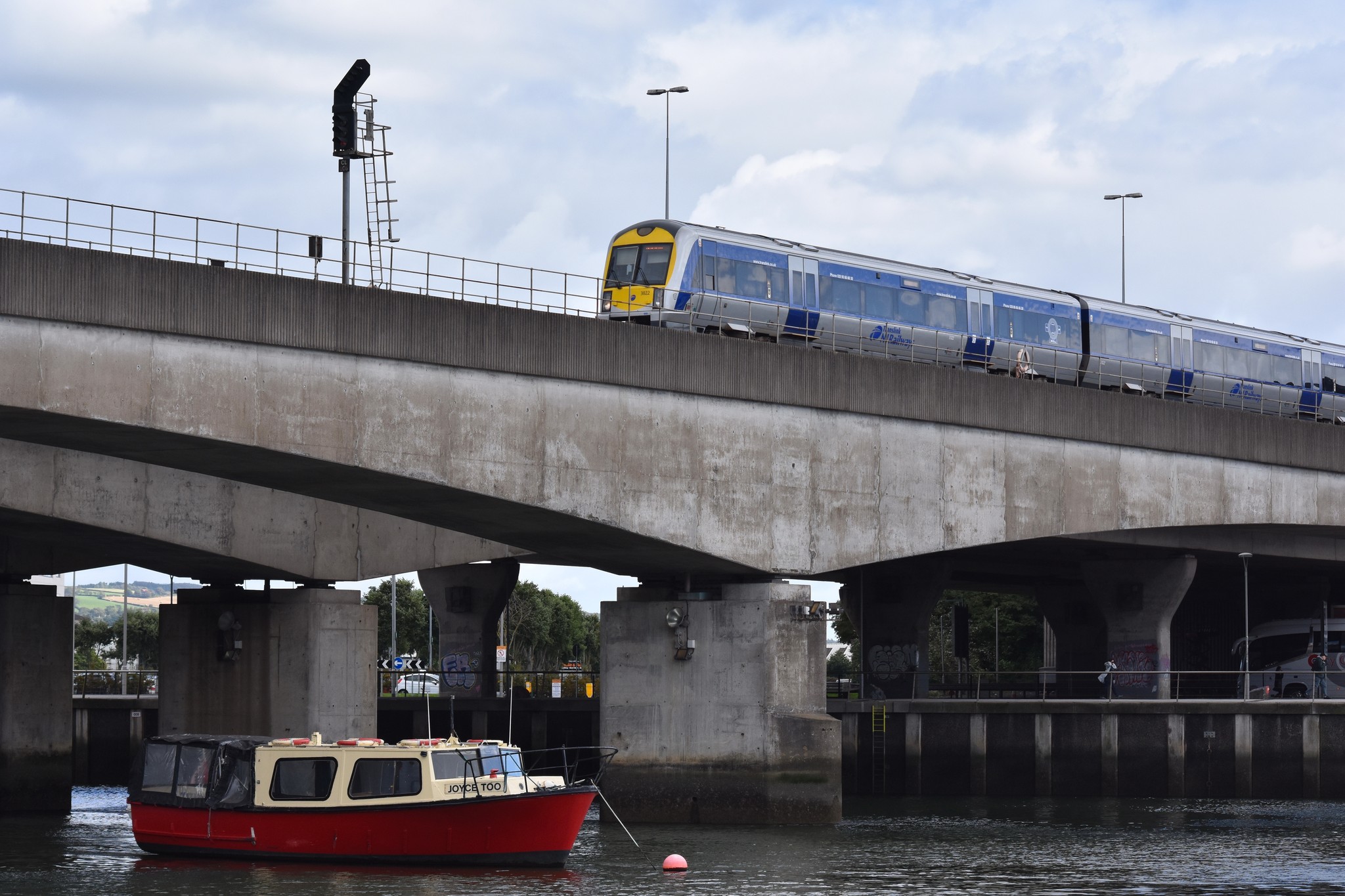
[1231,619,1345,700]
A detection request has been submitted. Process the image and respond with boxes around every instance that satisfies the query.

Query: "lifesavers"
[1016,349,1030,373]
[271,738,310,746]
[337,740,374,746]
[400,739,439,746]
[347,738,385,745]
[466,739,504,746]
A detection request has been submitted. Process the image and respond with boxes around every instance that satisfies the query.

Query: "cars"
[74,672,159,694]
[836,679,852,683]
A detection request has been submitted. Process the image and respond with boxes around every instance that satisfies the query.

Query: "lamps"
[828,602,841,620]
[810,602,824,620]
[218,611,243,632]
[666,606,690,629]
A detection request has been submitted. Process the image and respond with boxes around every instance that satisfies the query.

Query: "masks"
[1111,661,1114,664]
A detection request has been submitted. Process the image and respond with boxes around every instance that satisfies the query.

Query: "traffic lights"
[332,58,371,110]
[331,110,357,153]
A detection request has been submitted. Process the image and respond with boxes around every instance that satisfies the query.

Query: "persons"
[1272,666,1284,699]
[1304,652,1331,700]
[1098,659,1123,699]
[606,262,630,286]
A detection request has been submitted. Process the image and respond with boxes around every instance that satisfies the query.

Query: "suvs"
[393,673,440,693]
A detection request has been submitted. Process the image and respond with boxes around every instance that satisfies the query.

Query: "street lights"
[1102,194,1142,306]
[1237,551,1253,702]
[645,84,690,219]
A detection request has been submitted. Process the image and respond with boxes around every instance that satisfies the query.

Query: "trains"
[593,217,1345,432]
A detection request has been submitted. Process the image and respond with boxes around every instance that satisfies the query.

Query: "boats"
[121,682,620,871]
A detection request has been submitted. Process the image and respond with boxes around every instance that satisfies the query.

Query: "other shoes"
[1324,696,1331,699]
[1117,695,1123,699]
[1304,693,1310,699]
[1100,696,1107,699]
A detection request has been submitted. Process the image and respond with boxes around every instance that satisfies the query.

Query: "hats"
[1320,653,1328,657]
